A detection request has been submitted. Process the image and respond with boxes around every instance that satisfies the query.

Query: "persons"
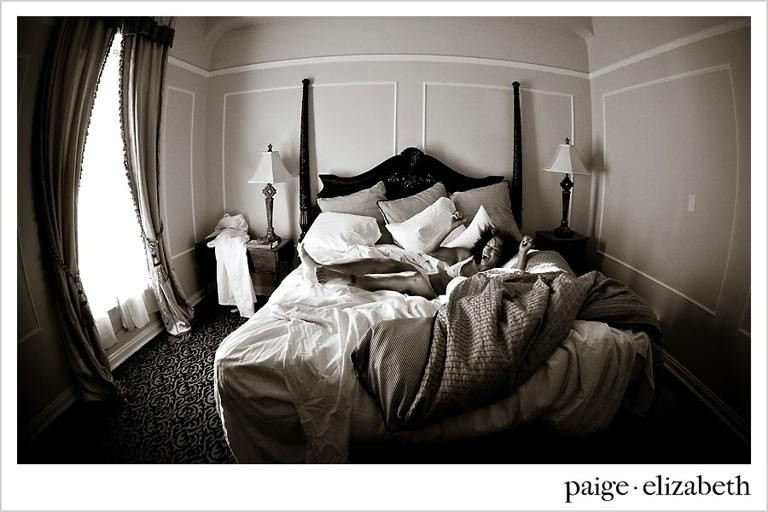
[297,223,537,301]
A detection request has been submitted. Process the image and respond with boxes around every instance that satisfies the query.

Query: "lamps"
[247,145,296,245]
[540,136,593,237]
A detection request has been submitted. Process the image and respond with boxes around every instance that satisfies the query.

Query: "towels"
[205,213,257,318]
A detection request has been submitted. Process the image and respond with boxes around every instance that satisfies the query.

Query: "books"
[243,237,279,250]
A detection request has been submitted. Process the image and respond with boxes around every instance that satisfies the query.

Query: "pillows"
[301,182,523,265]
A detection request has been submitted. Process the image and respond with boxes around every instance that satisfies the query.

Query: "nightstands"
[241,237,294,298]
[534,228,590,277]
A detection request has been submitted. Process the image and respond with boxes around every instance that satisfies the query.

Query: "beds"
[213,146,660,461]
